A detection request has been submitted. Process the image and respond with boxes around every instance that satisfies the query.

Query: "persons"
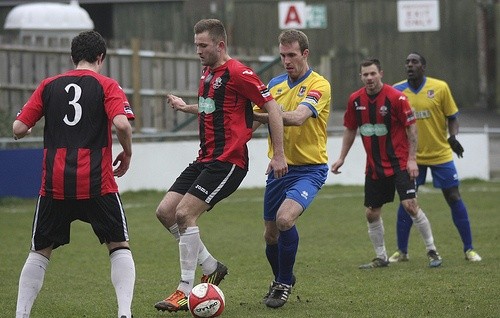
[382,52,483,265]
[153,17,290,312]
[331,58,446,269]
[12,31,145,318]
[239,29,328,309]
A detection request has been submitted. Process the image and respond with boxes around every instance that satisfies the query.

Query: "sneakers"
[200,261,229,287]
[154,290,189,312]
[359,257,389,269]
[263,280,277,304]
[389,250,408,262]
[265,275,296,309]
[464,248,481,262]
[426,249,442,268]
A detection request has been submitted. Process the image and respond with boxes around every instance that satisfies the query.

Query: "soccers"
[188,283,226,318]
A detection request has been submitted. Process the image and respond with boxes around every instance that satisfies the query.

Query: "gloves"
[448,135,464,158]
